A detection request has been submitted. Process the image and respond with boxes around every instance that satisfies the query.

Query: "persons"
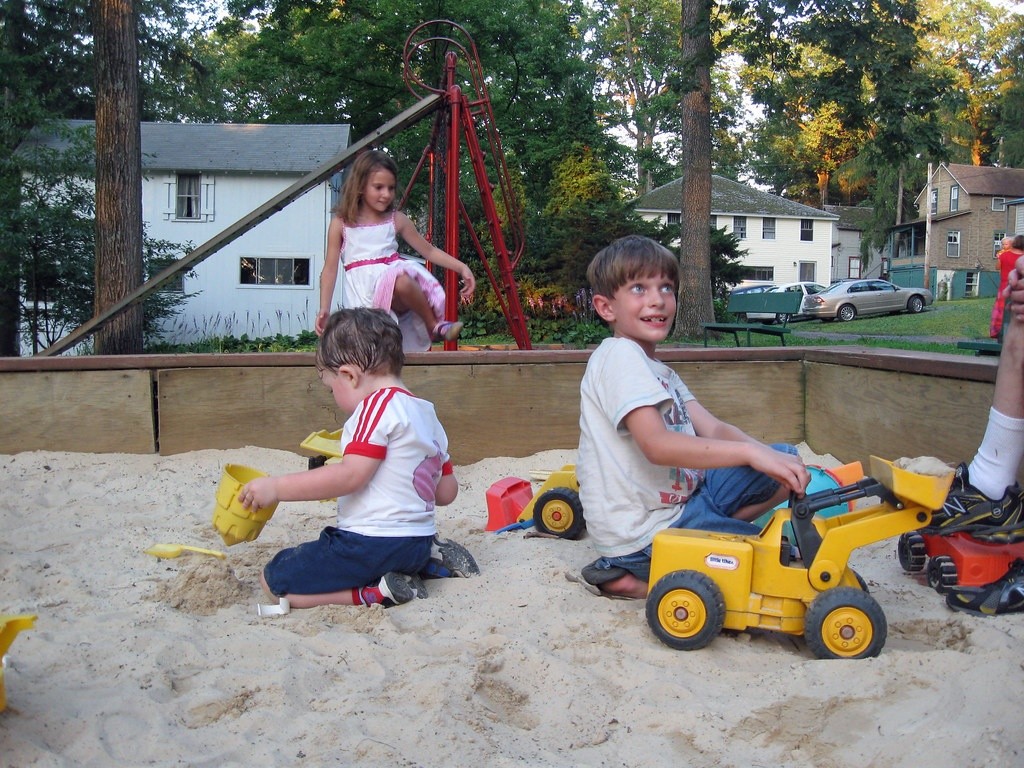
[315,148,477,351]
[913,234,1024,617]
[576,234,812,602]
[238,307,481,611]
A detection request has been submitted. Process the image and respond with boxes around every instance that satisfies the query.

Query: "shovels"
[144,543,225,560]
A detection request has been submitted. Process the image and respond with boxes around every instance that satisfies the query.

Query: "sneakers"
[429,321,463,343]
[361,572,427,609]
[429,533,479,578]
[947,558,1024,616]
[916,460,1024,542]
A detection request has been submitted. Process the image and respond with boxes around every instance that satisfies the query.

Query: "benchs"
[699,292,803,348]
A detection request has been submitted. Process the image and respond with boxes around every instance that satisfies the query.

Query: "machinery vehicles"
[641,454,958,661]
[483,465,587,542]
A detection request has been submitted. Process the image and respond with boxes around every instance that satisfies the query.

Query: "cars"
[728,283,777,295]
[803,279,934,322]
[746,281,828,323]
